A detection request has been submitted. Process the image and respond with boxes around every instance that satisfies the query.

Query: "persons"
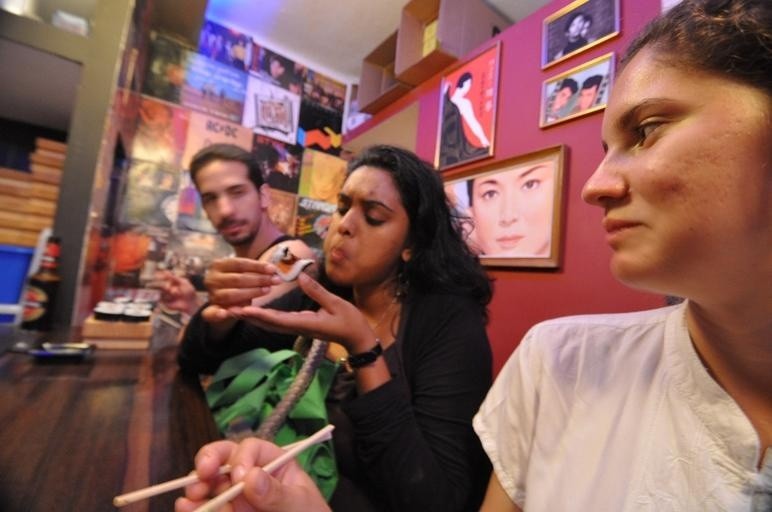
[463,1,772,512]
[551,12,602,61]
[436,74,490,170]
[195,22,347,111]
[175,146,492,510]
[545,78,578,123]
[453,158,559,259]
[572,76,602,117]
[144,141,331,350]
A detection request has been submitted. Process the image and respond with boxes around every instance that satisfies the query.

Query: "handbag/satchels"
[204,347,350,504]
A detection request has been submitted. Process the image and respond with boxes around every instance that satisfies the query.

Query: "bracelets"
[347,342,384,369]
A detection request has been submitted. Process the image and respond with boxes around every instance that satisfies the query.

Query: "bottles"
[18,234,63,337]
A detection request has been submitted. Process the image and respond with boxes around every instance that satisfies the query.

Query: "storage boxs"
[394,0,513,87]
[357,28,415,114]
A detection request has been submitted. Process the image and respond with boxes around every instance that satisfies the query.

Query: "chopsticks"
[111,422,338,512]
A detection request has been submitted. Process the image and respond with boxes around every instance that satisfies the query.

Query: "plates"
[26,339,94,358]
[89,299,154,322]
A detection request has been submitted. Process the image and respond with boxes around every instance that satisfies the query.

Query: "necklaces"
[350,286,402,330]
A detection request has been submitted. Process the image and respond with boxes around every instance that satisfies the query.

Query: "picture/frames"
[433,40,502,178]
[440,145,570,271]
[541,0,621,71]
[539,51,616,128]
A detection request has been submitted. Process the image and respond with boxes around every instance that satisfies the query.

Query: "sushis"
[272,246,314,283]
[93,297,156,322]
